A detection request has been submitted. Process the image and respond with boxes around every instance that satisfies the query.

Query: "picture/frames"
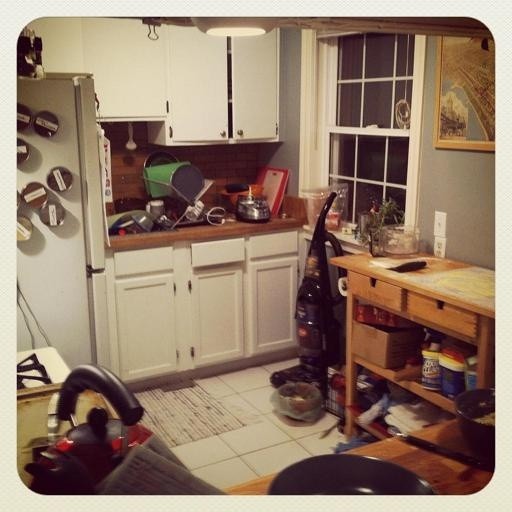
[432,36,496,153]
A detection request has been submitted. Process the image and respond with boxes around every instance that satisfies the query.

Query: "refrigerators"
[17,77,115,390]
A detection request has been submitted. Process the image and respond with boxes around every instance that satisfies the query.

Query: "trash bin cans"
[277,382,322,421]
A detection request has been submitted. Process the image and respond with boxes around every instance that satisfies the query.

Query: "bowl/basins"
[264,450,443,495]
[456,388,494,452]
[132,214,154,232]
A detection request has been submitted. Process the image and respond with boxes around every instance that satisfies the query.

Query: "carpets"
[129,376,246,448]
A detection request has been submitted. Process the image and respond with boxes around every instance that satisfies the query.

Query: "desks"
[220,419,495,494]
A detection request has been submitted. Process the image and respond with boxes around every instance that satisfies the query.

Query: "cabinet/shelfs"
[331,252,495,442]
[105,229,306,382]
[22,17,282,146]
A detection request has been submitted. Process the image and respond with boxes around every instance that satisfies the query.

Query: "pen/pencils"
[393,432,494,473]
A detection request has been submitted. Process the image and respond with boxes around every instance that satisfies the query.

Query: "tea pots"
[26,364,154,494]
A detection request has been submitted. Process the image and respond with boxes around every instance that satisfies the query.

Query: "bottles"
[150,201,165,220]
